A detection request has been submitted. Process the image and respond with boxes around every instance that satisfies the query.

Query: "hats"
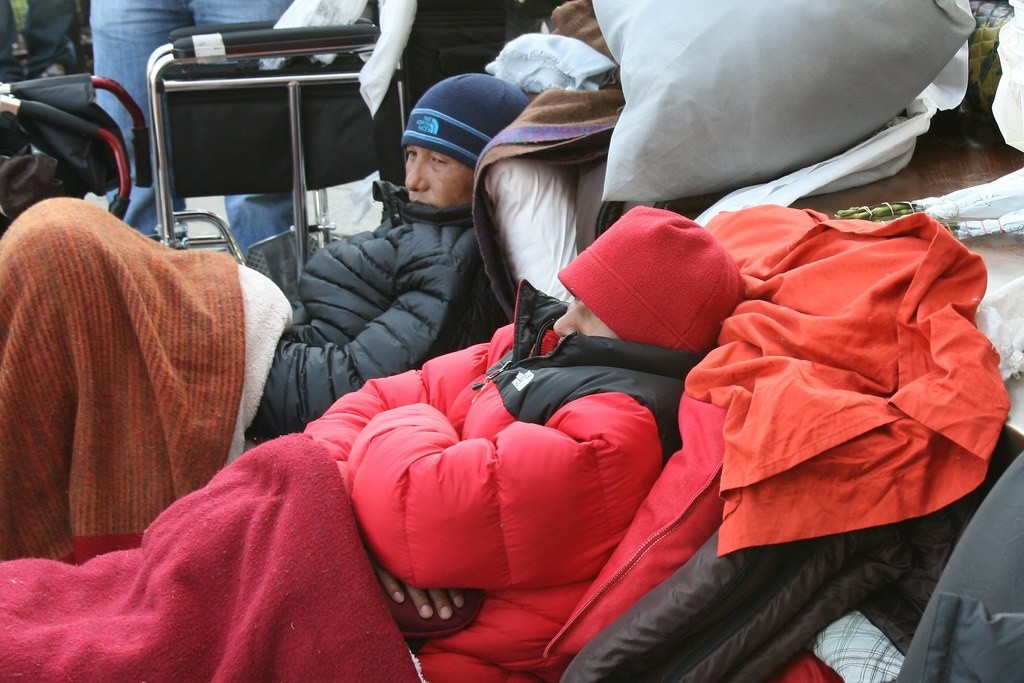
[558,205,745,351]
[401,73,532,172]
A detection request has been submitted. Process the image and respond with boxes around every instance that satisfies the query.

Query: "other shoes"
[39,63,65,78]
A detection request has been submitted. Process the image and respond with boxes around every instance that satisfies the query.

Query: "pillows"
[591,1,976,203]
[487,160,578,304]
[543,389,730,659]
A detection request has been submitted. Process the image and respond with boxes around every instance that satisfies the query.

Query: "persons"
[0,204,749,682]
[0,72,532,443]
[0,0,419,254]
